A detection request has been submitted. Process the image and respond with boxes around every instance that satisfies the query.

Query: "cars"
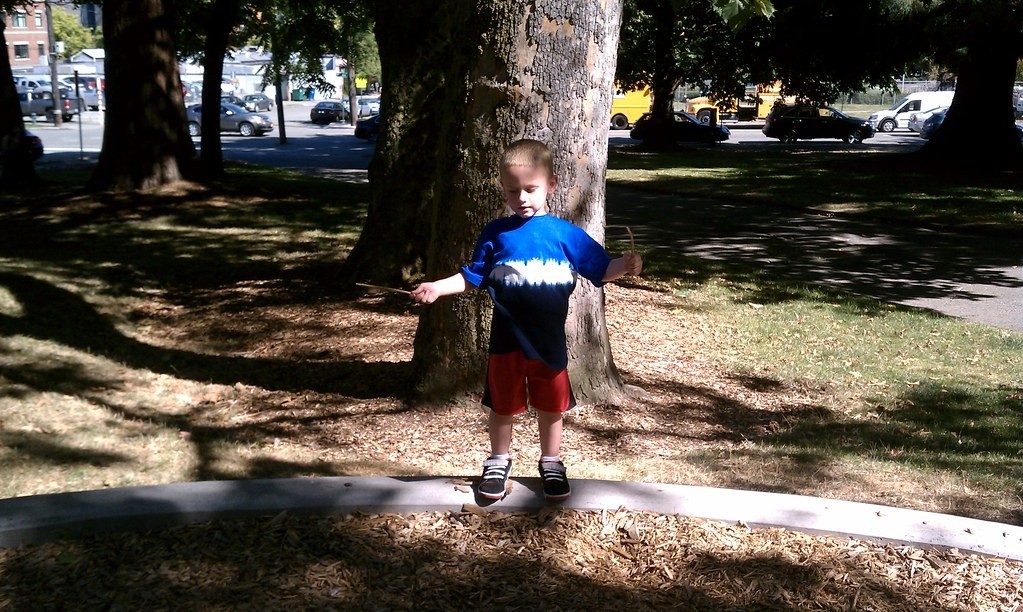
[220,94,247,110]
[908,106,950,133]
[920,107,949,139]
[13,72,106,124]
[181,75,244,104]
[630,110,730,144]
[763,98,876,145]
[241,93,274,114]
[186,100,274,137]
[311,101,350,124]
[341,92,381,119]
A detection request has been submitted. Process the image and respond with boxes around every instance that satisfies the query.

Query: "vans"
[868,91,955,133]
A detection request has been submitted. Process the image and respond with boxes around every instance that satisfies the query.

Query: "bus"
[609,77,655,130]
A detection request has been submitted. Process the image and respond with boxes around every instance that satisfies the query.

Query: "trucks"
[683,80,835,126]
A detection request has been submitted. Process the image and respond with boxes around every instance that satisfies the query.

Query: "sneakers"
[477,457,513,499]
[538,460,571,501]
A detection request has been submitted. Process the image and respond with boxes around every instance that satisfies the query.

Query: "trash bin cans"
[292,88,314,101]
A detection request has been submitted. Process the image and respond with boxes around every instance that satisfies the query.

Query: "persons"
[408,139,642,501]
